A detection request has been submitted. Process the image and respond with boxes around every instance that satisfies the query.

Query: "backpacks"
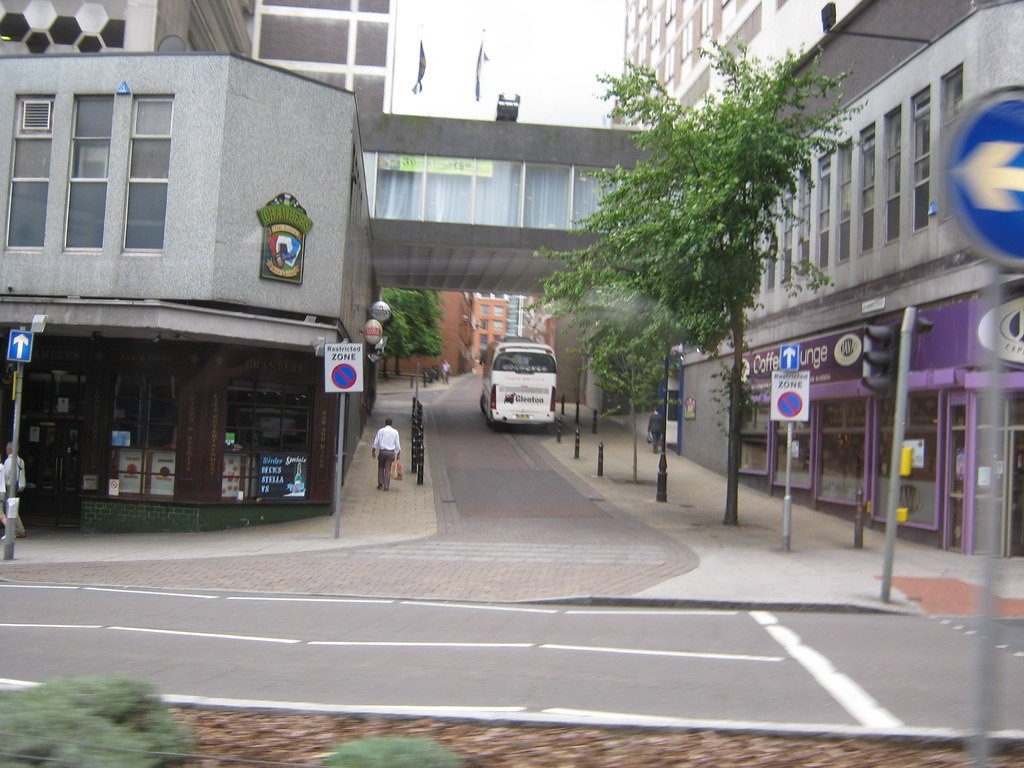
[16,458,26,492]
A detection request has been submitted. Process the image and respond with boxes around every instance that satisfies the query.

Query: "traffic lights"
[863,321,900,403]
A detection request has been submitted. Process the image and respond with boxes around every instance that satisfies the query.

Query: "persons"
[442,360,450,384]
[0,442,26,539]
[648,407,664,453]
[372,419,401,490]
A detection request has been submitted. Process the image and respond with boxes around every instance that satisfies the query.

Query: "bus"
[480,336,558,431]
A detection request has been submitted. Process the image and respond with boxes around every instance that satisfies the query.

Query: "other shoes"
[2,535,6,540]
[377,484,383,489]
[384,488,388,491]
[16,531,26,538]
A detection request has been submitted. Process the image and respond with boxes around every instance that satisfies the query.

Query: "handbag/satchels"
[391,456,404,480]
[647,431,652,443]
